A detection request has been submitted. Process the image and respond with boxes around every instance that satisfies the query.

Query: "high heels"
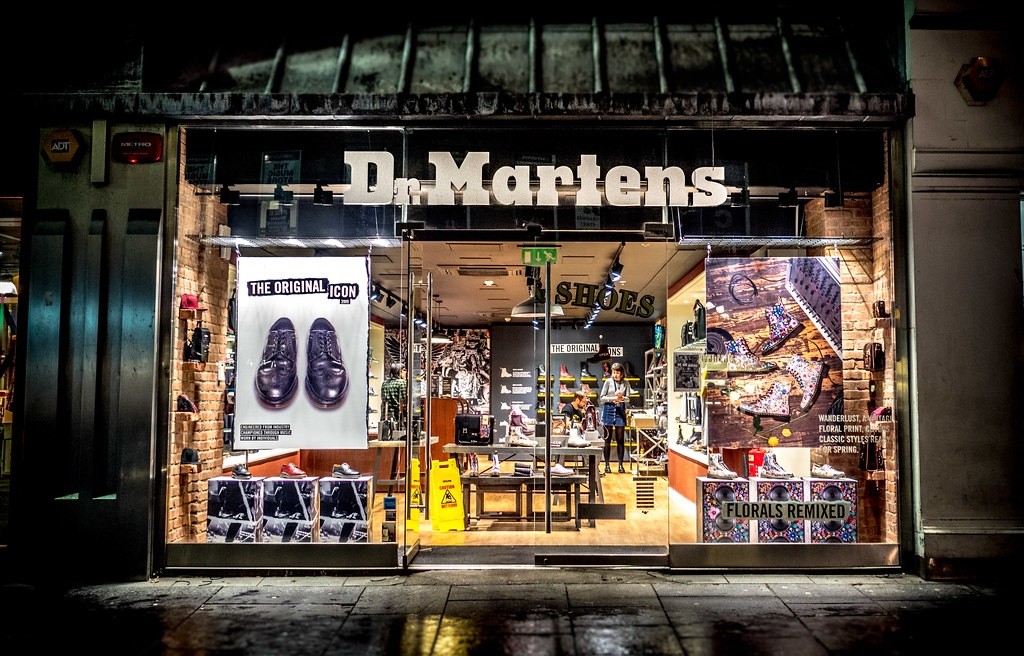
[685,427,702,445]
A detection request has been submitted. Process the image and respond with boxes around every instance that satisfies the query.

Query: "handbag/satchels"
[872,406,891,423]
[872,300,885,318]
[177,394,197,413]
[568,383,598,430]
[181,293,198,309]
[858,433,878,471]
[181,447,198,463]
[863,343,884,370]
[191,328,210,363]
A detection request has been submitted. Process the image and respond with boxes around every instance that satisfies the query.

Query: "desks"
[442,428,605,531]
[369,434,439,521]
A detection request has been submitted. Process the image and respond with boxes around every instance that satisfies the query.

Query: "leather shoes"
[544,463,575,478]
[333,462,360,479]
[231,463,252,479]
[280,462,309,479]
[584,469,606,478]
[306,318,349,411]
[253,317,299,411]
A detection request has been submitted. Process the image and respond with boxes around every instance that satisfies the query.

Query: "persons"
[381,362,407,420]
[599,363,631,473]
[561,391,586,421]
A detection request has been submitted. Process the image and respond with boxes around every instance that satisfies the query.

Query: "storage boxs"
[206,476,373,542]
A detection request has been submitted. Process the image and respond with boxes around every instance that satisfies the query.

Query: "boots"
[509,415,535,436]
[567,429,592,448]
[470,455,479,477]
[581,384,597,395]
[605,464,611,473]
[706,452,737,480]
[559,385,574,394]
[628,381,638,394]
[809,446,845,480]
[507,426,539,447]
[722,300,831,423]
[559,365,574,378]
[491,454,502,477]
[675,425,685,444]
[580,361,596,378]
[619,461,625,473]
[760,453,794,479]
[557,402,566,414]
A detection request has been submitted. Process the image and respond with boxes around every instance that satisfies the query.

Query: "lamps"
[825,193,844,209]
[584,263,624,329]
[779,191,798,208]
[420,330,455,344]
[730,190,749,208]
[369,285,436,334]
[313,187,334,206]
[273,186,294,206]
[953,56,1006,108]
[511,276,565,318]
[218,185,239,205]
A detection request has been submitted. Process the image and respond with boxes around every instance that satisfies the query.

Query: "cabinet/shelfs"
[628,345,669,476]
